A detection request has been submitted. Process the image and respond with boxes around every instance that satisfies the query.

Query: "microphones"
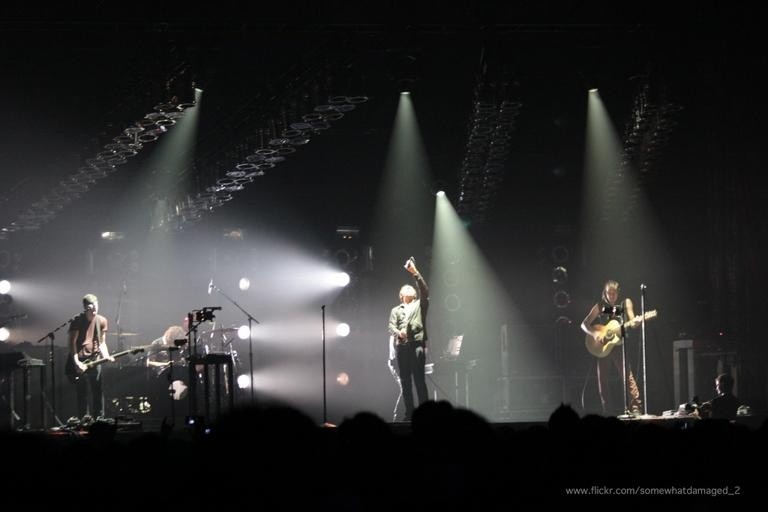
[87,304,93,312]
[208,278,213,294]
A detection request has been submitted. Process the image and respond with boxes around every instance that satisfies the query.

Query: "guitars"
[65,348,145,384]
[586,309,657,358]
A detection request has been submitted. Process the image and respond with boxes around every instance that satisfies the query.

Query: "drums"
[150,365,191,414]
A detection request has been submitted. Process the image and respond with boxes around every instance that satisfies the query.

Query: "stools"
[5,358,49,430]
[189,353,234,422]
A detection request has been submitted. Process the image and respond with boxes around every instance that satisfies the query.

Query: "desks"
[673,338,745,409]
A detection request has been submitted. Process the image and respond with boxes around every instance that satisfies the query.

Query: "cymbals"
[205,328,237,334]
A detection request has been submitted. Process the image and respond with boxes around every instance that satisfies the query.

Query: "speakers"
[500,322,558,375]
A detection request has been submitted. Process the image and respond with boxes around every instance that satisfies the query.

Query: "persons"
[688,373,737,423]
[580,278,643,415]
[386,334,406,423]
[66,292,117,420]
[145,325,204,413]
[387,257,430,422]
[0,397,768,512]
